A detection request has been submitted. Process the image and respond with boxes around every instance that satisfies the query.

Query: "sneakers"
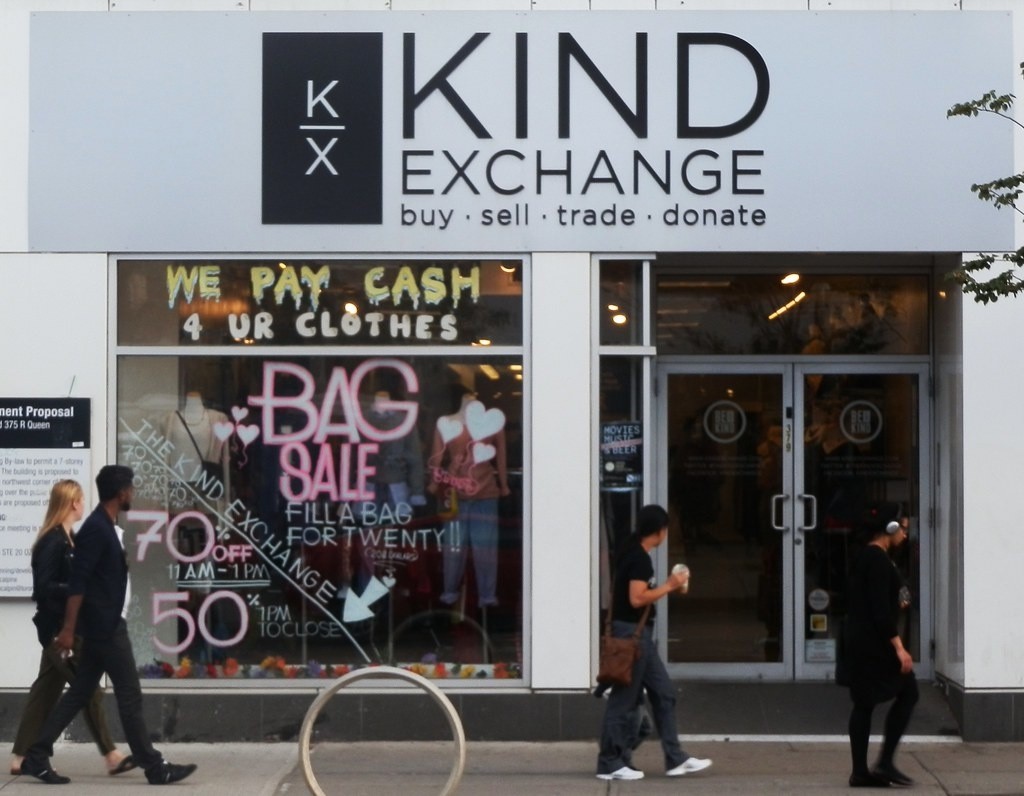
[595,766,645,781]
[666,756,712,775]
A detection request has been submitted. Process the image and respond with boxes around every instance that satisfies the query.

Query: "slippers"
[109,755,138,775]
[11,763,58,776]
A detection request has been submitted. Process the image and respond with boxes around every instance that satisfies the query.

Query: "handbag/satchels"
[596,634,639,686]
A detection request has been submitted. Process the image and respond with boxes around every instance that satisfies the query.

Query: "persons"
[160,391,233,678]
[674,414,719,550]
[430,391,510,607]
[756,445,831,665]
[347,391,428,520]
[11,465,198,785]
[832,505,918,787]
[592,505,713,781]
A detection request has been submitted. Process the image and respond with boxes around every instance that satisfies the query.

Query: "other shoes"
[21,757,70,784]
[871,756,913,786]
[146,760,197,786]
[849,769,891,787]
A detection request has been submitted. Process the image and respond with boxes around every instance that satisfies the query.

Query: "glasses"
[899,522,910,533]
